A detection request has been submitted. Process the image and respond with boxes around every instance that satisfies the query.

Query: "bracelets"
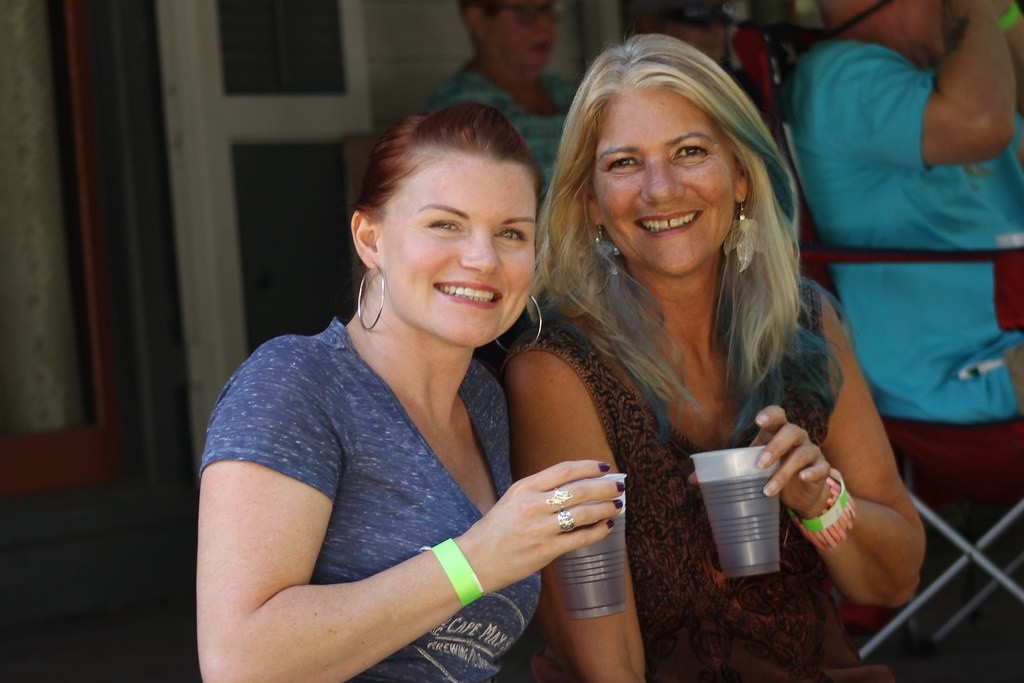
[418,537,484,607]
[788,459,856,552]
[996,0,1023,32]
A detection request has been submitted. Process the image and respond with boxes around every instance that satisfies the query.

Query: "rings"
[545,488,573,511]
[557,511,575,532]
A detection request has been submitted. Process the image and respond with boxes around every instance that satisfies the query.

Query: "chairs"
[733,23,1023,658]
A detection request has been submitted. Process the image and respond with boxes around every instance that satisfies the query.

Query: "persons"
[429,0,578,204]
[197,100,624,683]
[509,33,926,683]
[622,1,764,118]
[782,0,1024,479]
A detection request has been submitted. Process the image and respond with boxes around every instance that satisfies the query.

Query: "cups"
[548,473,629,620]
[689,445,781,578]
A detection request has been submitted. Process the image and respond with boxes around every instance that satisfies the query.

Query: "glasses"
[654,2,736,27]
[501,0,567,25]
